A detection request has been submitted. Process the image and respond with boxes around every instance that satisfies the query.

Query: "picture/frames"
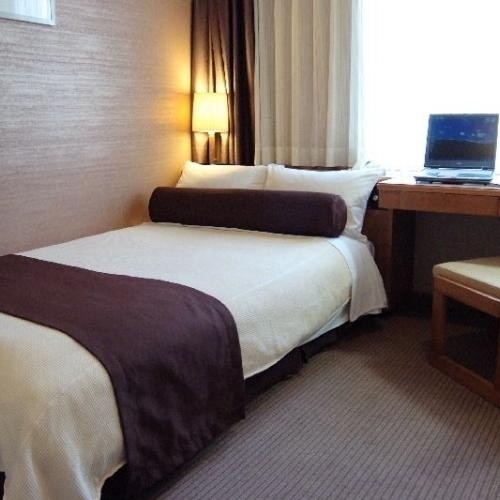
[0,1,55,26]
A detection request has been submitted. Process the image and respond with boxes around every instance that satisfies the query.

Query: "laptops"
[413,113,499,183]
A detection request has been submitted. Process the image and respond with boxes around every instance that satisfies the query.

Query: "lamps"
[192,92,229,165]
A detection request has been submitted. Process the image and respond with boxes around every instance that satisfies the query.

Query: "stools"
[431,255,500,408]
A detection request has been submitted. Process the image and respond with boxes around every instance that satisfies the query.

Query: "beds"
[1,161,388,499]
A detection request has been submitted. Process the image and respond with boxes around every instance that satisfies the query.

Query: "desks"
[377,175,500,281]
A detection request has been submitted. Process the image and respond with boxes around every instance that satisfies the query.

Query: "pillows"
[174,161,283,191]
[149,187,348,238]
[265,164,382,244]
